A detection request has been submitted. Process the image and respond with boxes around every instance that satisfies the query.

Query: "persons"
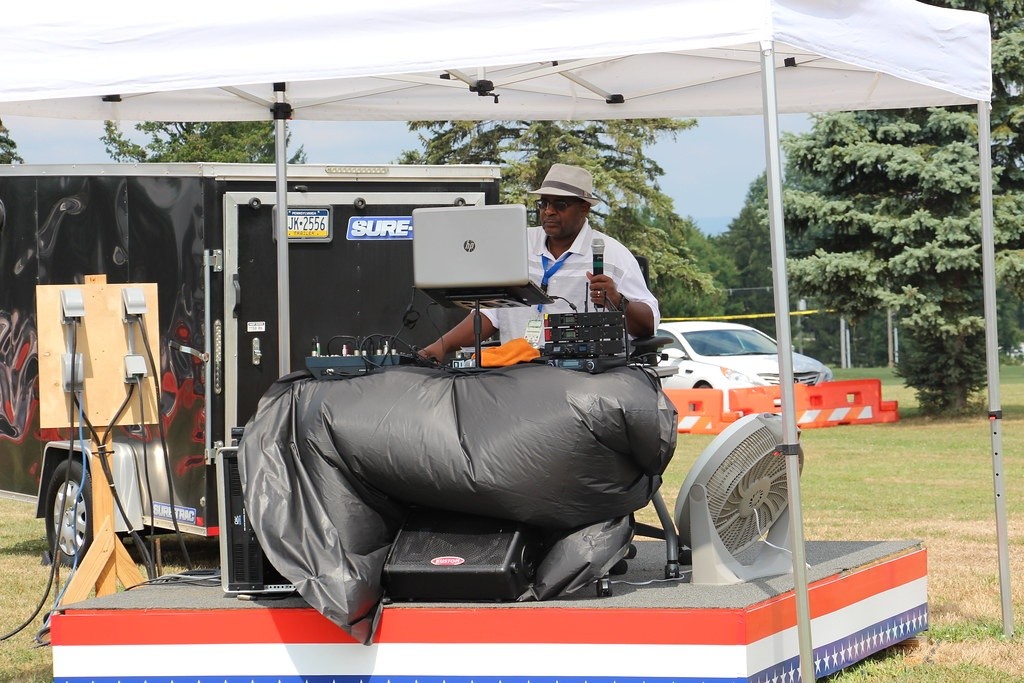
[418,163,661,365]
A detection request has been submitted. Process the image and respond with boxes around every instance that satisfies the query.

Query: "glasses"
[533,197,584,211]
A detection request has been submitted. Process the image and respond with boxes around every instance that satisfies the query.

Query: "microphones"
[591,238,605,307]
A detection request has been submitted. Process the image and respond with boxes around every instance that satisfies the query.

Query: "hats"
[528,161,601,206]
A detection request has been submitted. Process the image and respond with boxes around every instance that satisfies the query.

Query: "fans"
[670,412,807,587]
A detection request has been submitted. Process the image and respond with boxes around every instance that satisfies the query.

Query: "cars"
[653,319,832,391]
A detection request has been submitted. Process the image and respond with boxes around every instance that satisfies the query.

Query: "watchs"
[618,292,629,312]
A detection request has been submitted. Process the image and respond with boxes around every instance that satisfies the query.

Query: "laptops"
[412,203,555,305]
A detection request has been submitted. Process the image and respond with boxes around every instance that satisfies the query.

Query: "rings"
[597,290,600,297]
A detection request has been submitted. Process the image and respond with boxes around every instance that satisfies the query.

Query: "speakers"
[382,505,539,604]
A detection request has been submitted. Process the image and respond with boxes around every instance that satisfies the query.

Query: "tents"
[0,0,1015,683]
